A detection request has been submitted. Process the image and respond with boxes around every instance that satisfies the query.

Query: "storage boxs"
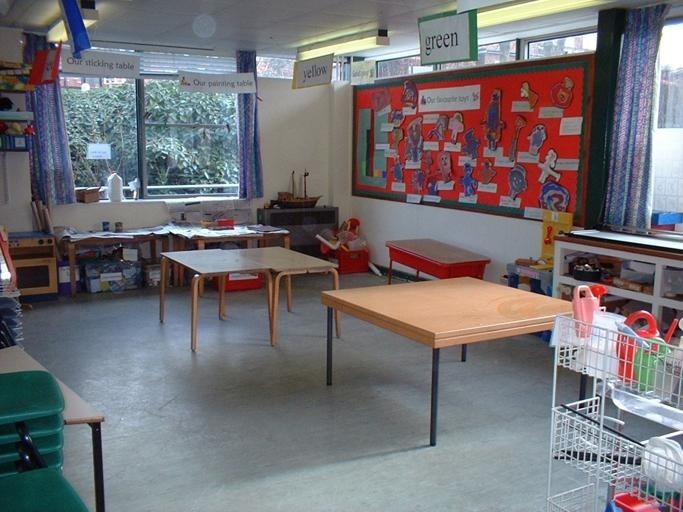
[224,272,263,291]
[335,244,368,274]
[74,187,101,203]
[140,261,172,290]
[57,260,81,296]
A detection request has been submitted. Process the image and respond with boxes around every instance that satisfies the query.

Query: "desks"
[159,248,273,352]
[223,246,340,346]
[177,229,290,287]
[60,233,178,299]
[0,344,106,512]
[321,276,586,446]
[385,239,490,285]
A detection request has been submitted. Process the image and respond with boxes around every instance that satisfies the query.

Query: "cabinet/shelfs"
[546,307,683,512]
[256,206,338,275]
[0,65,33,152]
[551,236,683,347]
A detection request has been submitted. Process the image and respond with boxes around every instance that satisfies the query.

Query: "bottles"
[103,222,110,232]
[115,222,123,233]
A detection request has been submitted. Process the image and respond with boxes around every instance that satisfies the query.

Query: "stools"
[505,263,551,338]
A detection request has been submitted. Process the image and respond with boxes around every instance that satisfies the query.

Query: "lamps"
[46,0,98,42]
[475,0,616,28]
[295,29,389,61]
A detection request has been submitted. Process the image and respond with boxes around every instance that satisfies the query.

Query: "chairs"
[0,370,90,512]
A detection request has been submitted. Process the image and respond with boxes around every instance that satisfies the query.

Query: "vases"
[107,173,123,202]
